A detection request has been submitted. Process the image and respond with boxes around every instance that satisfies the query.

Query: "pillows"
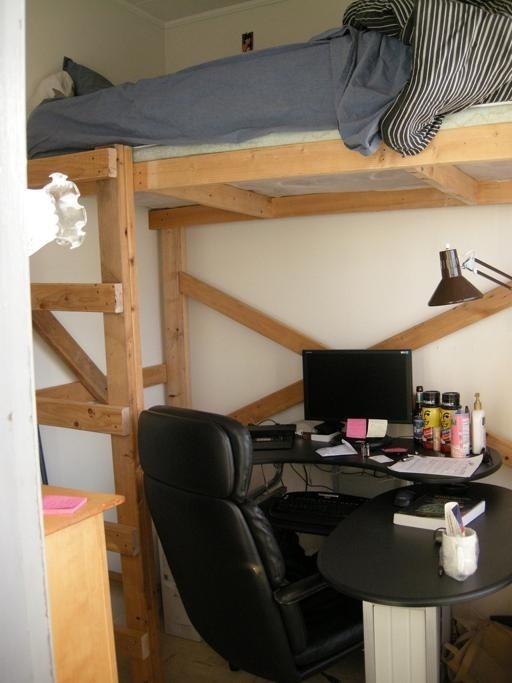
[62,56,114,96]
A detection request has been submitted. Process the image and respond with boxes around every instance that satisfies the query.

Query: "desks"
[42,484,127,683]
[248,435,512,682]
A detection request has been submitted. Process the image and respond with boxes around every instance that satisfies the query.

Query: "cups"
[450,412,470,457]
[440,528,480,579]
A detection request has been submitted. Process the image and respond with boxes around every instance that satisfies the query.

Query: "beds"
[27,122,512,682]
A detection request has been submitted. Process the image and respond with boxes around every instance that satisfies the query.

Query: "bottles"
[422,390,462,453]
[412,385,423,452]
[471,391,487,455]
[360,442,372,457]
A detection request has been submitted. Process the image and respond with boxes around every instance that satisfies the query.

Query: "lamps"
[429,244,512,306]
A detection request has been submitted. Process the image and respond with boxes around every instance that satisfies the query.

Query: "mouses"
[395,491,418,507]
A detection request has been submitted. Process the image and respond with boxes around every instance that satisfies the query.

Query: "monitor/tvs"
[302,349,412,448]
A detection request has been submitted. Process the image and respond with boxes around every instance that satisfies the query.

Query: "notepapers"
[42,494,87,514]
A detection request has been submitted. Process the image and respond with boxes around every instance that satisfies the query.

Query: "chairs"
[138,405,363,682]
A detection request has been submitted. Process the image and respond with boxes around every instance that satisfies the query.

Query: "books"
[393,491,486,532]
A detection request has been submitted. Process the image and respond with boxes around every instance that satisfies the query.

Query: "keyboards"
[268,491,370,526]
